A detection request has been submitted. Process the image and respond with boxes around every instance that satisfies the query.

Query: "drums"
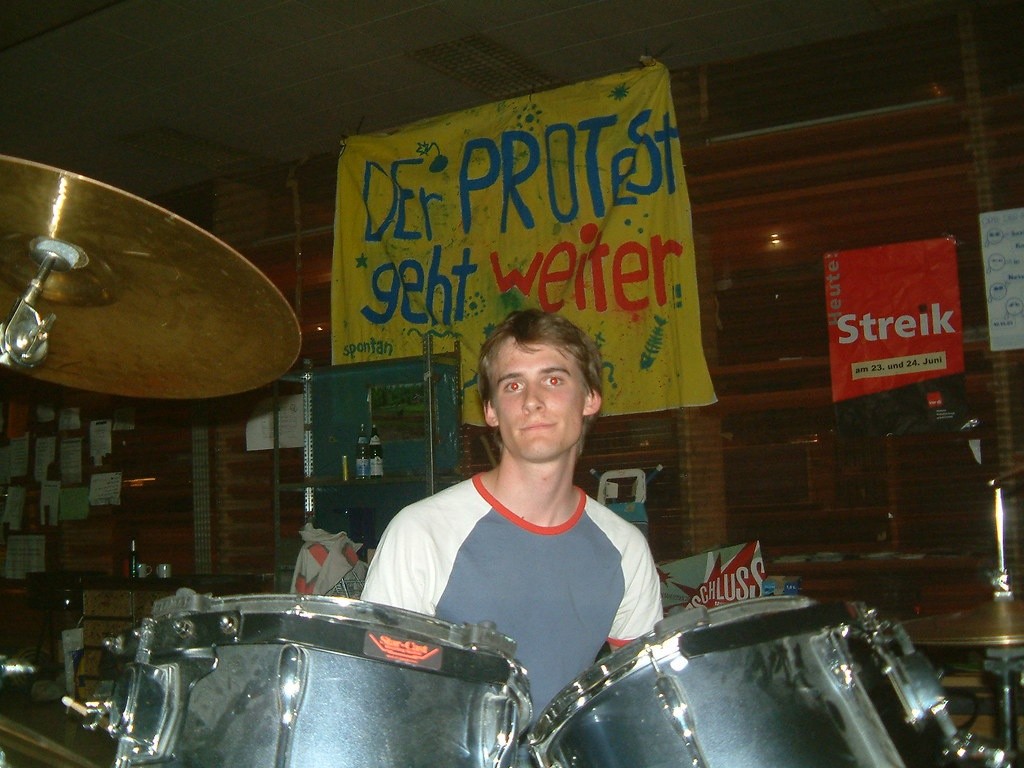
[110,590,537,768]
[528,591,993,768]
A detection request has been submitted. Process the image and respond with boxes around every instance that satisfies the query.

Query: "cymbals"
[1,155,301,399]
[882,595,1024,647]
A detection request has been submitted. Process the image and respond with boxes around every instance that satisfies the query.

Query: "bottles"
[355,424,369,480]
[368,425,383,478]
[127,538,138,581]
[762,580,775,597]
[784,581,800,596]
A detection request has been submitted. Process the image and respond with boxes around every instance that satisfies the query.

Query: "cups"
[156,563,172,579]
[136,563,152,578]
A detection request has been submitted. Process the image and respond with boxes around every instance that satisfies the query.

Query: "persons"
[359,307,663,768]
